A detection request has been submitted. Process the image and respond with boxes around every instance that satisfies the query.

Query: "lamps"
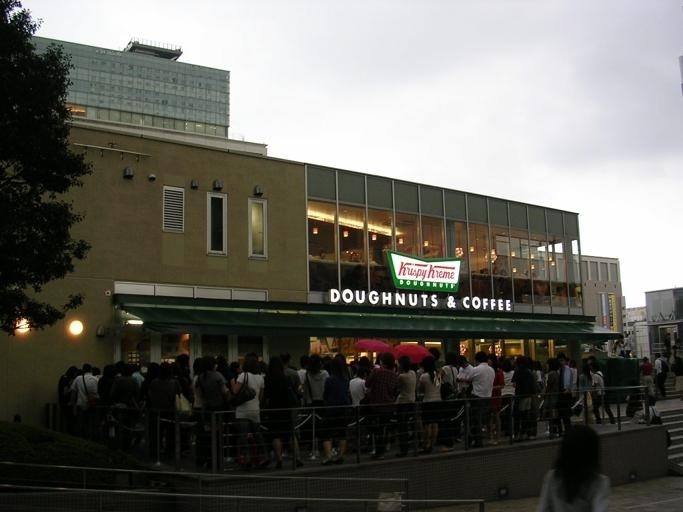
[312,220,555,272]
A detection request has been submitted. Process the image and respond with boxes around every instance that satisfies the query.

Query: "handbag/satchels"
[652,416,663,425]
[440,383,456,399]
[174,393,193,418]
[312,399,326,418]
[228,382,257,409]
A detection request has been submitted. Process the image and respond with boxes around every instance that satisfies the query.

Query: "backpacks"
[659,358,669,374]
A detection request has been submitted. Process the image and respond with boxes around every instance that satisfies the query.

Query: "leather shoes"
[272,432,562,471]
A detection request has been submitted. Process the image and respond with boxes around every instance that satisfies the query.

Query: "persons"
[56,350,616,470]
[652,352,666,396]
[638,356,655,398]
[615,337,624,357]
[664,332,670,358]
[668,345,680,386]
[622,333,631,358]
[638,392,659,424]
[318,247,326,260]
[535,421,612,511]
